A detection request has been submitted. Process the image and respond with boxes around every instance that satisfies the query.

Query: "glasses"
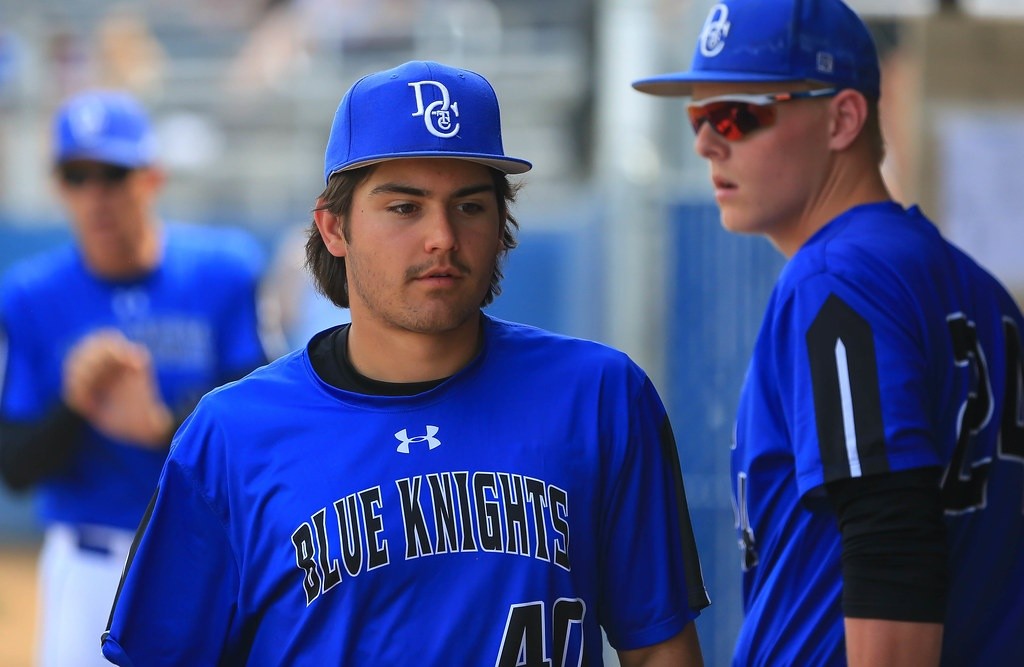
[686,87,839,141]
[63,167,132,188]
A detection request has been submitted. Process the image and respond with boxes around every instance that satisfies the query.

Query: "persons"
[0,96,270,667]
[94,57,711,667]
[631,0,1024,667]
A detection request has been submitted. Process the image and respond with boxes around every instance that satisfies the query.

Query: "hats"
[49,88,159,170]
[324,60,533,187]
[632,1,881,98]
